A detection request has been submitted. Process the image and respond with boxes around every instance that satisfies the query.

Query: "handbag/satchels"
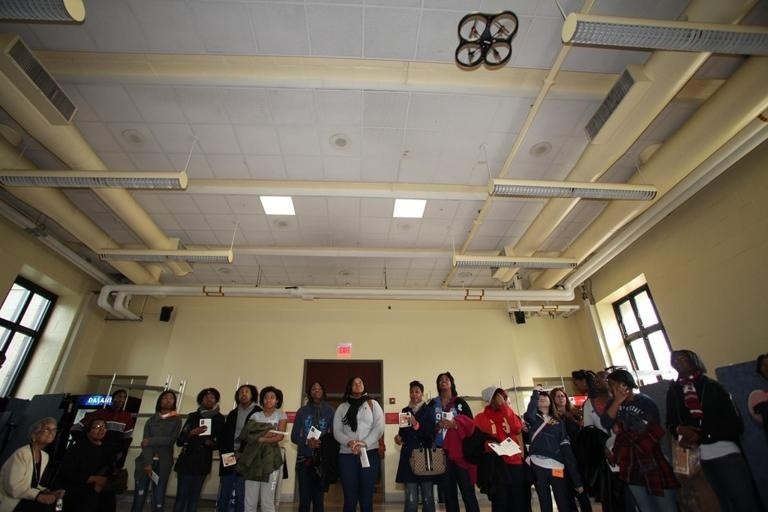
[407,435,448,477]
[374,430,385,457]
[671,434,702,478]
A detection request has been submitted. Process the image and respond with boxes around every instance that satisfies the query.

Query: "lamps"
[555,1,768,56]
[98,222,239,264]
[0,136,198,191]
[452,238,577,269]
[480,144,658,201]
[0,0,86,23]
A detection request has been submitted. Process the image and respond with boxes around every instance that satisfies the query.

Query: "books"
[148,470,160,487]
[306,425,322,441]
[198,418,212,437]
[262,429,288,440]
[221,452,237,467]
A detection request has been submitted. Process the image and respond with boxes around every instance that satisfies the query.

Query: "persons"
[0,414,66,512]
[130,390,183,512]
[393,347,768,512]
[332,375,386,512]
[290,379,336,500]
[172,387,226,512]
[242,386,288,512]
[216,384,264,512]
[68,389,134,470]
[61,417,118,512]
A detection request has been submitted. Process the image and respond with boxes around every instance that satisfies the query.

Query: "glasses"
[38,427,57,433]
[90,424,106,430]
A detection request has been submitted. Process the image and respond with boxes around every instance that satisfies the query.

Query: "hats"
[480,385,505,407]
